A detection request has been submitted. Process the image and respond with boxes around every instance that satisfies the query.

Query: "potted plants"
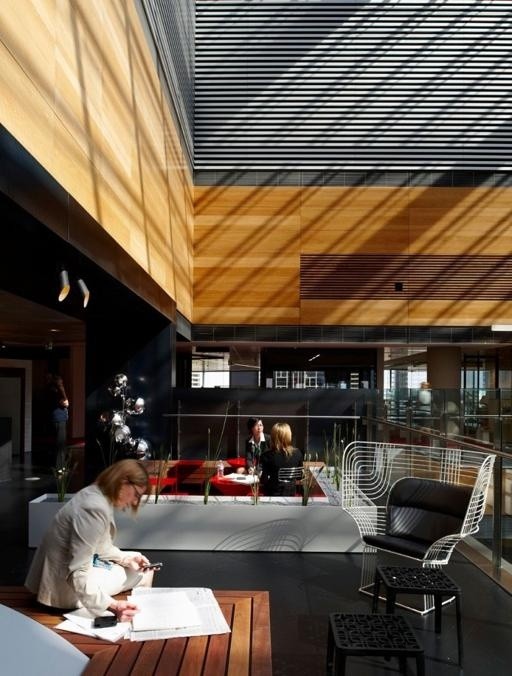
[26,400,381,556]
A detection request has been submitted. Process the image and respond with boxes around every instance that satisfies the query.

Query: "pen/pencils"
[126,605,134,629]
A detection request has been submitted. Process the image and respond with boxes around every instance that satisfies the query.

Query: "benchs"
[0,583,274,675]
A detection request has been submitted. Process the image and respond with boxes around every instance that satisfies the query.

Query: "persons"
[245,415,271,475]
[24,457,151,623]
[257,422,302,495]
[40,363,70,477]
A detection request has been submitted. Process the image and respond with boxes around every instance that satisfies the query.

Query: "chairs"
[146,456,324,496]
[342,438,497,616]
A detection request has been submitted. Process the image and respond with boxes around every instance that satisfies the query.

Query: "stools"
[371,565,467,667]
[324,608,426,675]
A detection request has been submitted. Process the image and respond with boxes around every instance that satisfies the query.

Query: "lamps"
[55,270,72,303]
[75,278,91,310]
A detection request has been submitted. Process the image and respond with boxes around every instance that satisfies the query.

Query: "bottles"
[216,460,225,478]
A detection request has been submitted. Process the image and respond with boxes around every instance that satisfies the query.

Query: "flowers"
[95,373,153,469]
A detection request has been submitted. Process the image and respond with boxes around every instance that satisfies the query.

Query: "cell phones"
[142,562,163,569]
